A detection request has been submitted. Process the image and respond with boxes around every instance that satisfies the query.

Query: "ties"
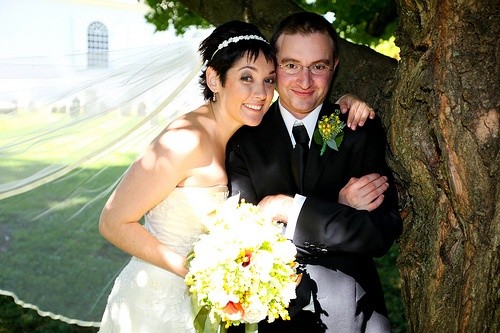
[291,125,311,194]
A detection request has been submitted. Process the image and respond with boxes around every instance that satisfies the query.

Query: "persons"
[97,22,375,333]
[225,12,400,333]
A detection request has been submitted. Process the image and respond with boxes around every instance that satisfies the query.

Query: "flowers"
[184,191,303,333]
[314,110,346,157]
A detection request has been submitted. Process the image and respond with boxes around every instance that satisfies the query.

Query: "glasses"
[276,62,335,75]
